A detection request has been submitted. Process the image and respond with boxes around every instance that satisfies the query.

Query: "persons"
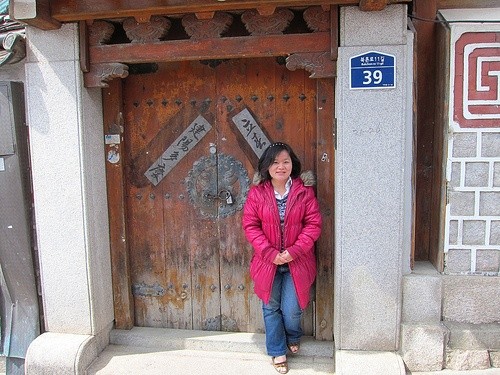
[241,142,322,374]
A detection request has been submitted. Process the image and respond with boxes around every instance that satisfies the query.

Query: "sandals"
[288,342,299,353]
[272,356,288,374]
